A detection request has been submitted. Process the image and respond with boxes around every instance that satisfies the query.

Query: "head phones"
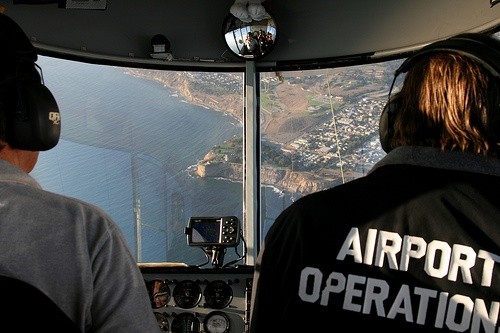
[0,12,62,151]
[378,37,500,152]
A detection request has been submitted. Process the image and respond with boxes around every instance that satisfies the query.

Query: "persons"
[248,33,500,333]
[0,12,162,333]
[245,29,274,54]
